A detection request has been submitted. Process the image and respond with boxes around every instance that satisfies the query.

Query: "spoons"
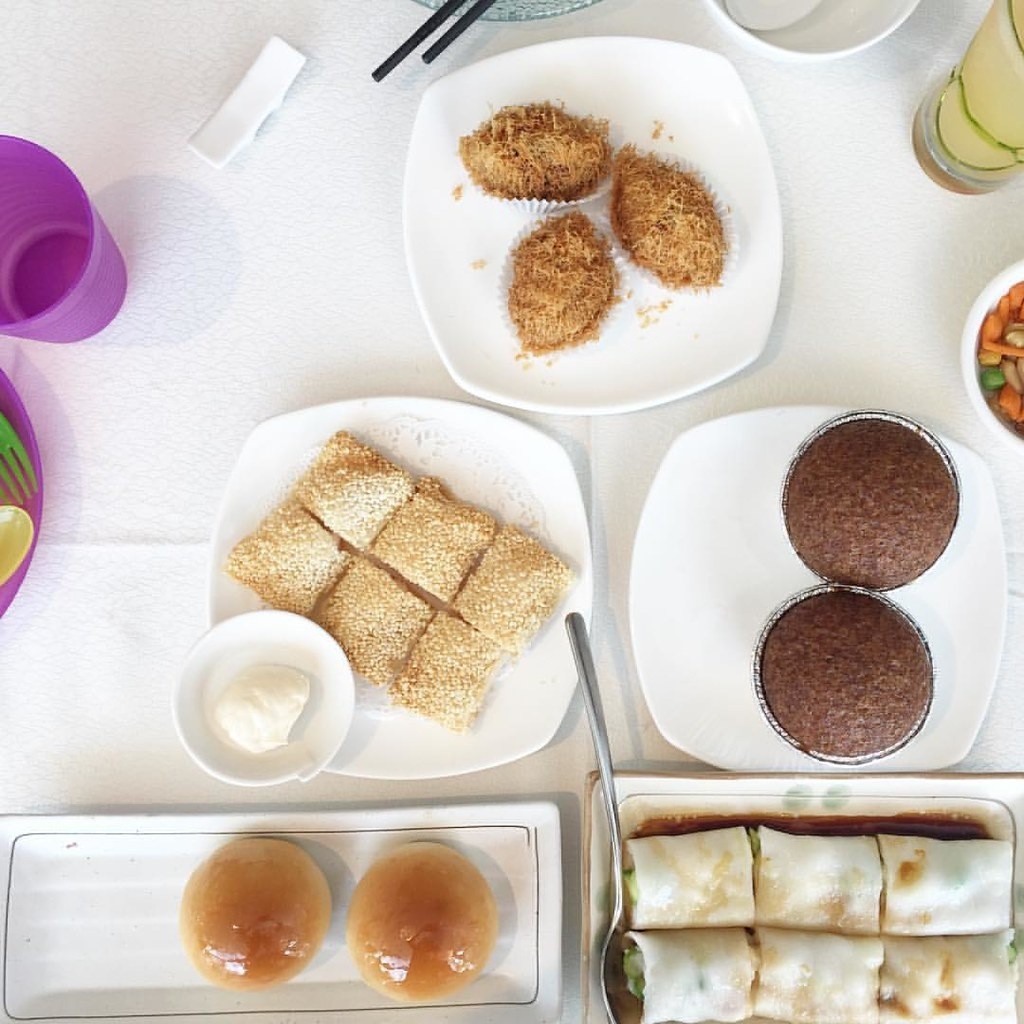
[728,0,814,37]
[0,505,34,582]
[565,611,643,1024]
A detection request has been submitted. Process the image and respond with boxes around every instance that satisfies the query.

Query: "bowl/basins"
[712,0,922,80]
[959,255,1024,455]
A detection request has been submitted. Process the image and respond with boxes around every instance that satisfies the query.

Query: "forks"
[0,413,37,506]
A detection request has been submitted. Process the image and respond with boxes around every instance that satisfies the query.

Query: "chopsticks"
[370,0,495,84]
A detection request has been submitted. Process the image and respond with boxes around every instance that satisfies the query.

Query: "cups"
[1,135,128,345]
[913,0,1024,198]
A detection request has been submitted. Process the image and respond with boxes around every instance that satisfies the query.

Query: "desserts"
[459,99,727,359]
[754,407,961,767]
[343,841,497,1002]
[177,840,333,993]
[224,430,574,737]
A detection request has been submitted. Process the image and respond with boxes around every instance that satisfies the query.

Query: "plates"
[170,610,358,786]
[0,365,44,620]
[419,1,599,25]
[633,404,1009,773]
[405,36,784,415]
[0,800,561,1023]
[214,395,594,780]
[580,769,1024,1024]
